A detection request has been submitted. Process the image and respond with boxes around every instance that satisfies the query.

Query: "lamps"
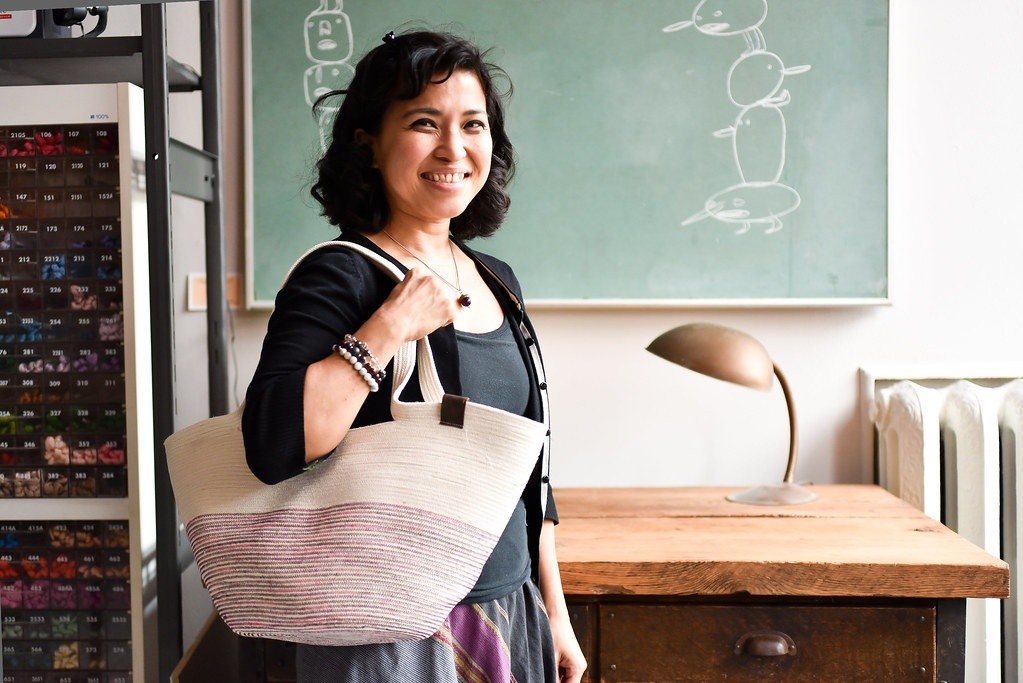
[641,317,824,512]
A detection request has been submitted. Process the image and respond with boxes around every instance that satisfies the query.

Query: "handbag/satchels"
[163,240,547,646]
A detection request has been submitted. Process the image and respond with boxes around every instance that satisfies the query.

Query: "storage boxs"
[0,122,133,683]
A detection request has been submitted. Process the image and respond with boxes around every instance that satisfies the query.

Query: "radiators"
[855,370,1023,683]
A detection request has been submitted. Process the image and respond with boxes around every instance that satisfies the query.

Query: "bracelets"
[332,334,386,392]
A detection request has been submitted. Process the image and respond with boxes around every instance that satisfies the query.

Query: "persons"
[242,31,588,683]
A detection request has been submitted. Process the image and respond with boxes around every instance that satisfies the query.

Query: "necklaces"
[382,228,472,306]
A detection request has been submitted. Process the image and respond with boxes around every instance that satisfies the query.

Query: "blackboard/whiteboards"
[243,1,894,312]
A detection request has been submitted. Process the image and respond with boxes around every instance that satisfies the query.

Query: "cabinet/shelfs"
[0,83,161,683]
[545,476,1012,683]
[0,0,232,683]
